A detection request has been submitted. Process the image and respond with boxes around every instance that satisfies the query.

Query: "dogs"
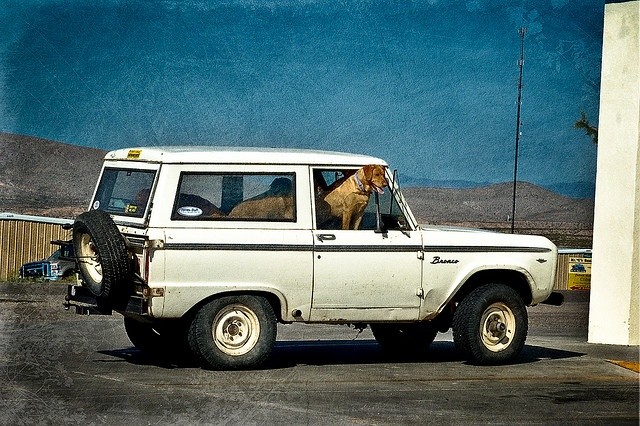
[136,188,228,220]
[228,177,296,221]
[309,164,389,230]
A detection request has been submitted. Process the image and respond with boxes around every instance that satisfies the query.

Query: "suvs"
[48,144,560,366]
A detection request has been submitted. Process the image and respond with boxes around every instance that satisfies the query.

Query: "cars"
[19,244,78,284]
